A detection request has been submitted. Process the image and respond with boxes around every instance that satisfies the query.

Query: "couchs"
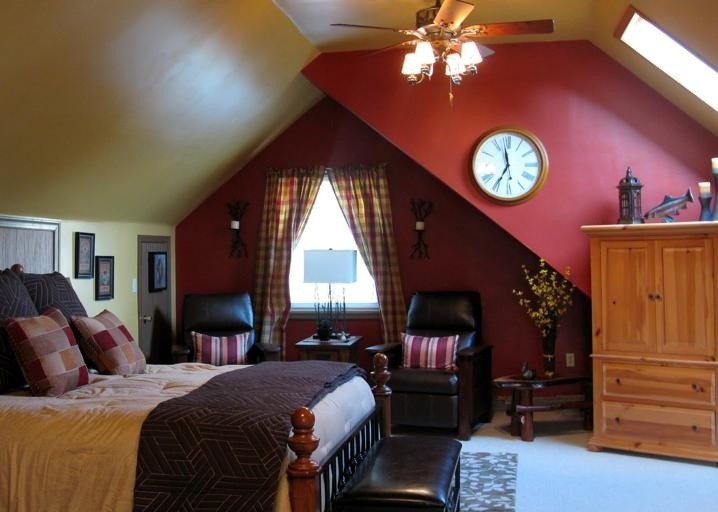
[364,290,493,441]
[171,291,281,363]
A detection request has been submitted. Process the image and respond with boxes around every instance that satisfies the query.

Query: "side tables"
[296,335,364,362]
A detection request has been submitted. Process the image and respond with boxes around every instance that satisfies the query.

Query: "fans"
[328,1,554,61]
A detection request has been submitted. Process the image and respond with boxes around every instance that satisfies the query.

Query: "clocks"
[466,124,549,207]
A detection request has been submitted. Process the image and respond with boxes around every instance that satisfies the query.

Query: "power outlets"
[565,353,575,367]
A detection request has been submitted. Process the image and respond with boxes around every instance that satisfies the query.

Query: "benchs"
[335,435,462,511]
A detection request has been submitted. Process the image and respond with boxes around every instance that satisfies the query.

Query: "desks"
[493,374,591,441]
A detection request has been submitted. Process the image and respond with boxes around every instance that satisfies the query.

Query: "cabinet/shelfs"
[579,221,718,464]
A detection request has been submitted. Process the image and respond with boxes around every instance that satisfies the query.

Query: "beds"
[0,264,392,511]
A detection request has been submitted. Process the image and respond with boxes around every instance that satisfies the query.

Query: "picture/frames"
[148,252,168,292]
[75,232,95,279]
[95,255,114,301]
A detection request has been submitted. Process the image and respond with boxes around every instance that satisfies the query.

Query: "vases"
[544,341,555,378]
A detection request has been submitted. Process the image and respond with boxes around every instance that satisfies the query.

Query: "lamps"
[402,1,484,86]
[304,247,357,340]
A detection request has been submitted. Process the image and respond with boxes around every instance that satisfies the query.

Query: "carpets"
[459,451,519,511]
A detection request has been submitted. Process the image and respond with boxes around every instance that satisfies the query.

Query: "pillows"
[17,272,88,333]
[69,309,146,376]
[191,331,249,367]
[0,267,39,395]
[398,332,459,370]
[1,306,89,396]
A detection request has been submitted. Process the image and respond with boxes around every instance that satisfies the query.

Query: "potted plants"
[317,318,332,341]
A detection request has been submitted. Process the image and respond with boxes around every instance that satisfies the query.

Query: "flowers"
[511,258,576,340]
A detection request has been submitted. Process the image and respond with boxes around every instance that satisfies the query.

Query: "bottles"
[340,327,346,342]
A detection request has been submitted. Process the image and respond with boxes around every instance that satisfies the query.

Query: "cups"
[318,320,331,341]
